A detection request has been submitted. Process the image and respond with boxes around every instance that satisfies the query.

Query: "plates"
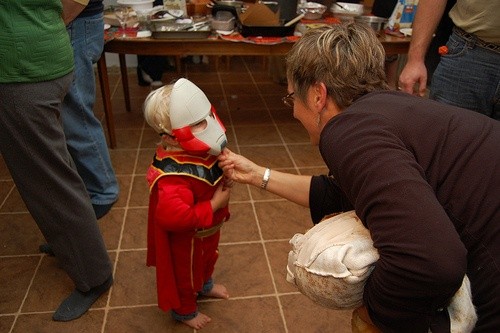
[399,28,436,37]
[103,24,111,31]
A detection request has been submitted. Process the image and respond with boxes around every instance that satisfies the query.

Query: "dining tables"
[97,31,411,148]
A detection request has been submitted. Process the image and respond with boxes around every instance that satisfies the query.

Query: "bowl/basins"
[152,18,177,26]
[354,16,389,37]
[298,6,327,19]
[331,10,360,19]
[117,0,154,11]
[206,0,244,18]
[211,17,234,31]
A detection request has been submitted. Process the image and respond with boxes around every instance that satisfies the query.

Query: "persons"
[56,0,119,221]
[398,0,500,122]
[142,77,232,330]
[218,19,500,333]
[0,0,115,323]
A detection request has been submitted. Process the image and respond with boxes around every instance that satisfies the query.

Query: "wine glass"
[114,7,128,38]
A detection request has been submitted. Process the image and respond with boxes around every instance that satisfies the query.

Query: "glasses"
[281,92,298,106]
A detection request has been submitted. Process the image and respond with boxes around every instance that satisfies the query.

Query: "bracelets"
[260,167,271,189]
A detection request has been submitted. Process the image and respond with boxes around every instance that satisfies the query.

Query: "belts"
[453,26,500,53]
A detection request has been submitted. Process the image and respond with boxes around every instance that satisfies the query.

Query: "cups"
[216,12,232,21]
